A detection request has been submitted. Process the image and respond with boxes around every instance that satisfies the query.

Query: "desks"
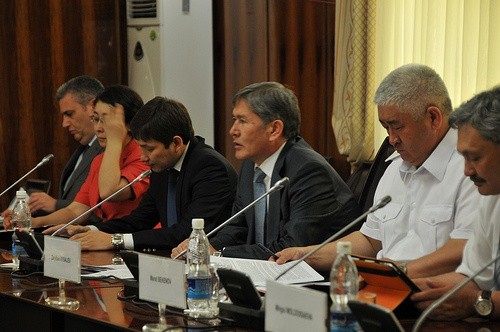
[0,247,500,332]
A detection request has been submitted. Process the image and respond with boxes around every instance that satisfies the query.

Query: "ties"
[252,168,267,246]
[166,168,178,228]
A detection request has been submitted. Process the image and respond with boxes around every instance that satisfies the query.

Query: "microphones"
[174,177,288,260]
[52,169,152,236]
[0,153,54,196]
[272,196,392,282]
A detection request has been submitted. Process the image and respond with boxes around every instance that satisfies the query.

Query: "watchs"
[112,251,123,265]
[111,233,124,250]
[474,289,493,319]
[214,250,222,256]
[398,262,407,276]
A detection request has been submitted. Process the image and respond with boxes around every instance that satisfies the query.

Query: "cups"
[209,264,219,318]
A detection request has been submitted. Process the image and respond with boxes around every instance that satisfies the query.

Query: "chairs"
[344,136,393,233]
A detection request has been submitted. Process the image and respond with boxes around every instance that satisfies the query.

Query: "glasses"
[88,115,105,127]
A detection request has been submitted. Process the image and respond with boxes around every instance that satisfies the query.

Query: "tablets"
[348,254,422,318]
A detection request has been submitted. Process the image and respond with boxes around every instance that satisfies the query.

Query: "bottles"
[329,241,362,332]
[186,218,211,312]
[10,190,32,269]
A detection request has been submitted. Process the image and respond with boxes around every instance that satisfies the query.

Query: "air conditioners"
[126,0,214,150]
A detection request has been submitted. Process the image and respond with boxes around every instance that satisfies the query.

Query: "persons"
[409,87,500,322]
[41,97,238,257]
[171,82,355,260]
[3,84,162,230]
[268,62,477,279]
